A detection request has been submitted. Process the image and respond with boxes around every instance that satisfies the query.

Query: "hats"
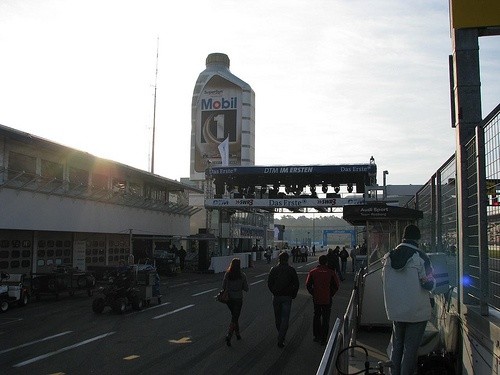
[402,224,421,240]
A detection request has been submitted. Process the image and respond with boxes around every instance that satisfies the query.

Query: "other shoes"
[277,343,286,349]
[313,337,320,342]
[320,339,327,345]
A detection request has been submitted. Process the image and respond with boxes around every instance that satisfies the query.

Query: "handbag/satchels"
[214,289,230,304]
[417,352,458,375]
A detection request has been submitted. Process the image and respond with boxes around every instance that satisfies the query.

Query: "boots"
[224,323,236,348]
[233,321,242,341]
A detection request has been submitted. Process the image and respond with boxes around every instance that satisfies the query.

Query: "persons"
[312,245,315,256]
[170,244,187,274]
[381,224,436,375]
[268,252,299,348]
[327,246,349,282]
[291,245,308,263]
[306,255,339,346]
[221,257,249,346]
[350,246,357,275]
[253,245,279,264]
[450,243,456,257]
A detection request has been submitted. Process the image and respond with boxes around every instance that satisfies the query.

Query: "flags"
[218,138,228,166]
